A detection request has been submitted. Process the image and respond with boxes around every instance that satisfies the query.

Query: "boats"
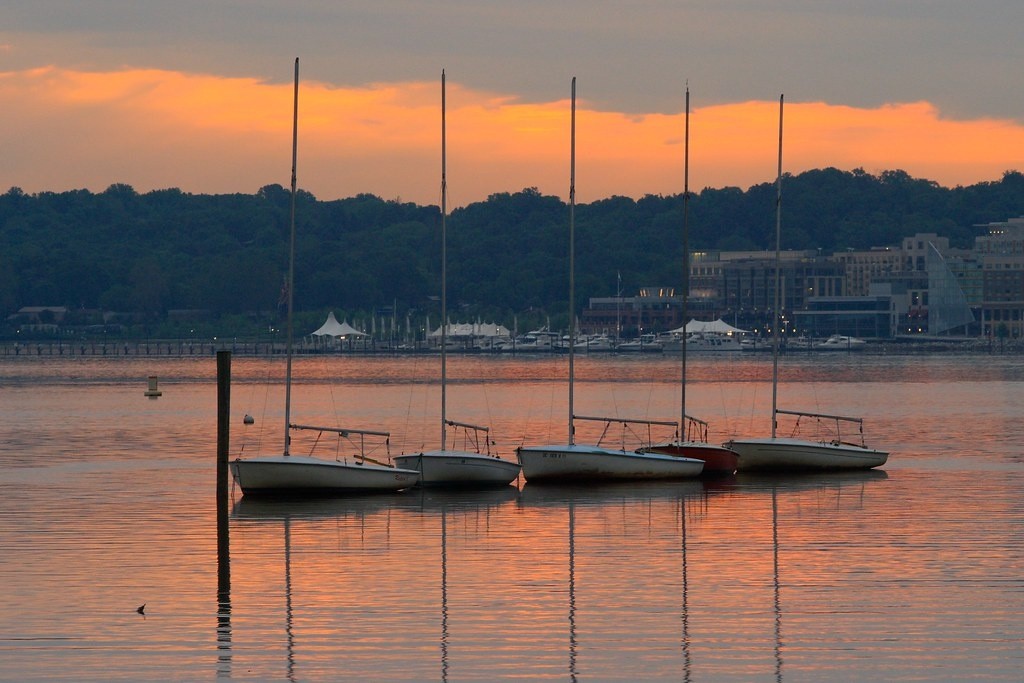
[662,334,739,351]
[473,338,515,351]
[516,337,555,350]
[819,335,866,349]
[395,343,415,350]
[574,338,614,351]
[741,336,814,350]
[642,339,673,350]
[615,334,655,351]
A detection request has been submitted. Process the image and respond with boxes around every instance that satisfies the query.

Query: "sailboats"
[723,94,891,472]
[513,76,706,482]
[636,86,741,473]
[393,70,522,487]
[226,57,420,495]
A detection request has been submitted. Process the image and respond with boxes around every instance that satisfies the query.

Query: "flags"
[618,271,621,281]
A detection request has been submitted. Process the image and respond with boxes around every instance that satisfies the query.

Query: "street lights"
[781,328,785,343]
[754,328,758,348]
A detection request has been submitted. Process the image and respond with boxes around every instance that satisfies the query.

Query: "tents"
[664,318,753,334]
[431,322,510,336]
[312,311,369,338]
